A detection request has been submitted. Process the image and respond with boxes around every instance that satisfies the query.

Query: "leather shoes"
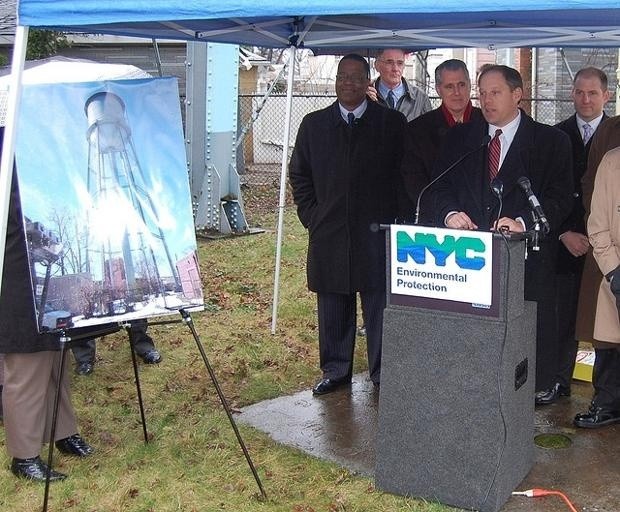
[575,398,620,429]
[533,382,571,403]
[136,347,163,365]
[56,433,98,457]
[76,359,94,375]
[313,377,352,395]
[11,457,67,485]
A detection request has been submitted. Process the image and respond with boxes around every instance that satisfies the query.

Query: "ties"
[347,113,355,128]
[488,129,502,185]
[386,90,395,109]
[583,123,592,147]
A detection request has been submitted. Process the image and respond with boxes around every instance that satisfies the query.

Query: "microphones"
[413,135,491,226]
[516,175,547,225]
[489,181,504,232]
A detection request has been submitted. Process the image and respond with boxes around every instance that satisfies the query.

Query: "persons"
[402,59,481,223]
[0,127,92,482]
[288,54,409,393]
[70,317,162,377]
[366,48,433,123]
[535,67,611,405]
[587,144,620,344]
[422,65,573,360]
[574,115,619,430]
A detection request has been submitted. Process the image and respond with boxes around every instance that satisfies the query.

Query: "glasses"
[336,73,369,83]
